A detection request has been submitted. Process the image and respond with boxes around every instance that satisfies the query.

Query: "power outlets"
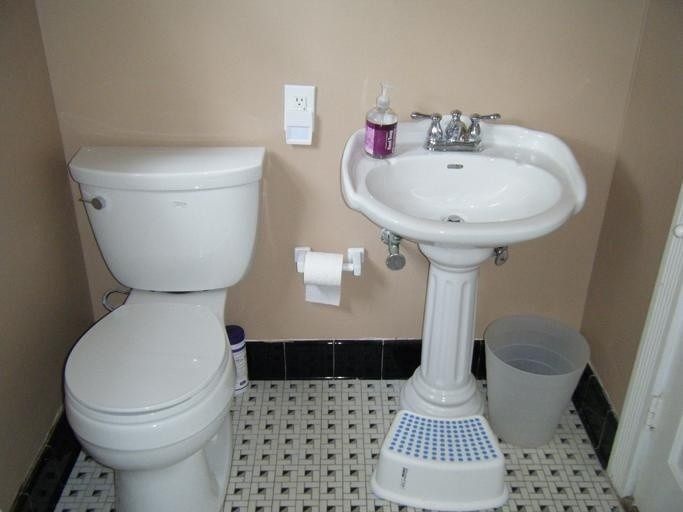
[283,83,317,132]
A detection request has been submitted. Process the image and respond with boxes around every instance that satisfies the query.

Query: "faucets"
[444,110,466,143]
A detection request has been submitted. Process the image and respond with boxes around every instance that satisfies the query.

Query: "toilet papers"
[302,251,343,309]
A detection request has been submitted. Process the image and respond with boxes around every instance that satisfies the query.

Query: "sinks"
[339,112,587,250]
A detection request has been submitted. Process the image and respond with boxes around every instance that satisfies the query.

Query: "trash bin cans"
[484,314,591,448]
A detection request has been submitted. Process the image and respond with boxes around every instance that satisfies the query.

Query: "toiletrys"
[363,81,399,160]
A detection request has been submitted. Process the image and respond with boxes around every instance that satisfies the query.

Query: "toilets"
[65,144,266,512]
